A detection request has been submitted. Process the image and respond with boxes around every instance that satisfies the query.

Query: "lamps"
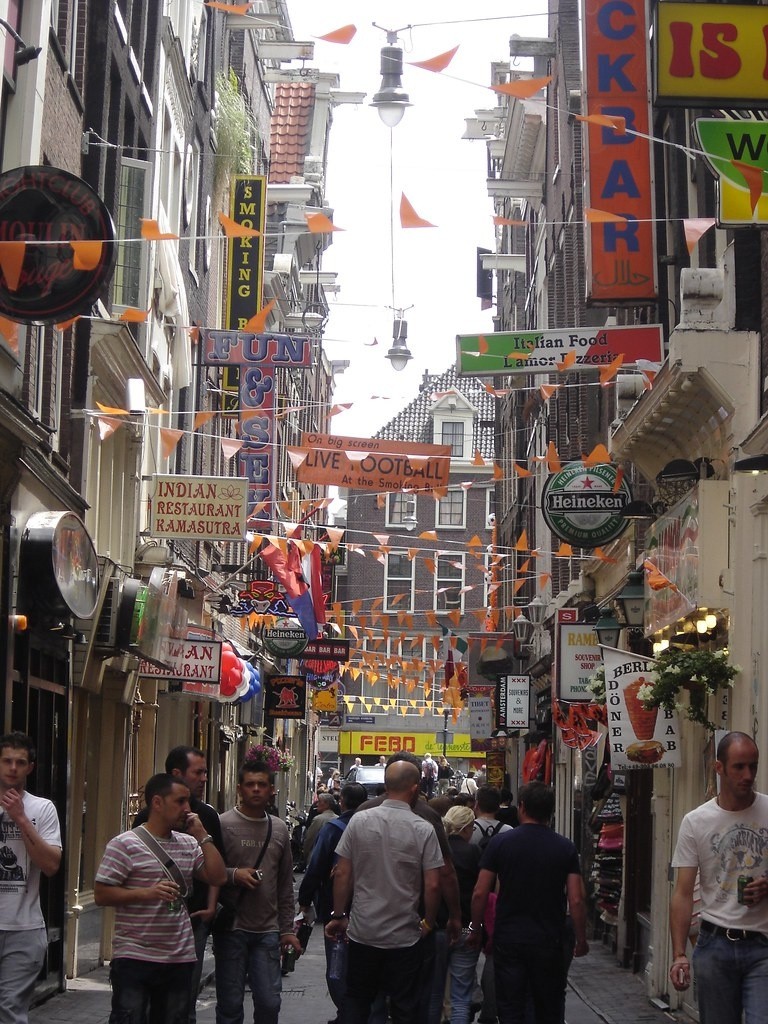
[732,453,768,476]
[370,22,412,130]
[528,594,550,639]
[512,611,538,650]
[614,572,645,631]
[238,723,267,737]
[0,18,42,66]
[582,603,601,622]
[401,501,418,532]
[618,500,666,519]
[652,615,717,651]
[177,556,232,614]
[385,305,413,371]
[591,609,622,658]
[62,624,88,644]
[655,457,716,484]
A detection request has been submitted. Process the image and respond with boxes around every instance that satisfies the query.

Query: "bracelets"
[420,919,432,932]
[673,953,686,962]
[469,922,483,933]
[330,910,345,920]
[198,835,212,846]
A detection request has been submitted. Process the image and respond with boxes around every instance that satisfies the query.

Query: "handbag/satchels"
[210,896,234,933]
[297,921,313,955]
[444,767,454,778]
[482,891,498,958]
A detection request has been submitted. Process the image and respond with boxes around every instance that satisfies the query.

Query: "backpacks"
[474,821,504,850]
[423,761,435,777]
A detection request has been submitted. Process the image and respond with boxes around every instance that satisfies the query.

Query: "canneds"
[168,885,181,911]
[281,949,295,972]
[737,875,754,905]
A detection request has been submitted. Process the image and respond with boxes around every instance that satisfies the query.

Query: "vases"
[683,680,706,691]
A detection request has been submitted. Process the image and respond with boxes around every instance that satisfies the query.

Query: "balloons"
[221,641,261,703]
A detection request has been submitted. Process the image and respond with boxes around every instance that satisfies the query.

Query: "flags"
[259,525,327,641]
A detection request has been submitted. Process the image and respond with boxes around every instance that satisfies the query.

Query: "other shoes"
[328,1017,339,1024]
[477,1016,498,1024]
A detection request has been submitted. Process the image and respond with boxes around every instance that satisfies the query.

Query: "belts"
[700,919,760,941]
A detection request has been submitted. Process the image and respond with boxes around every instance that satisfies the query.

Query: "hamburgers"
[626,741,664,764]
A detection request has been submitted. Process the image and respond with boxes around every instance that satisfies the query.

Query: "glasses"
[470,827,476,831]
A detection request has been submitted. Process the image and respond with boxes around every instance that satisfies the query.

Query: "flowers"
[244,745,296,774]
[636,647,742,729]
[586,664,605,702]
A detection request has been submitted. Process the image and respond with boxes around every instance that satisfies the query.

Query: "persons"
[132,745,223,1024]
[298,748,591,1024]
[93,773,228,1024]
[0,731,63,1024]
[669,731,768,1024]
[217,745,302,1023]
